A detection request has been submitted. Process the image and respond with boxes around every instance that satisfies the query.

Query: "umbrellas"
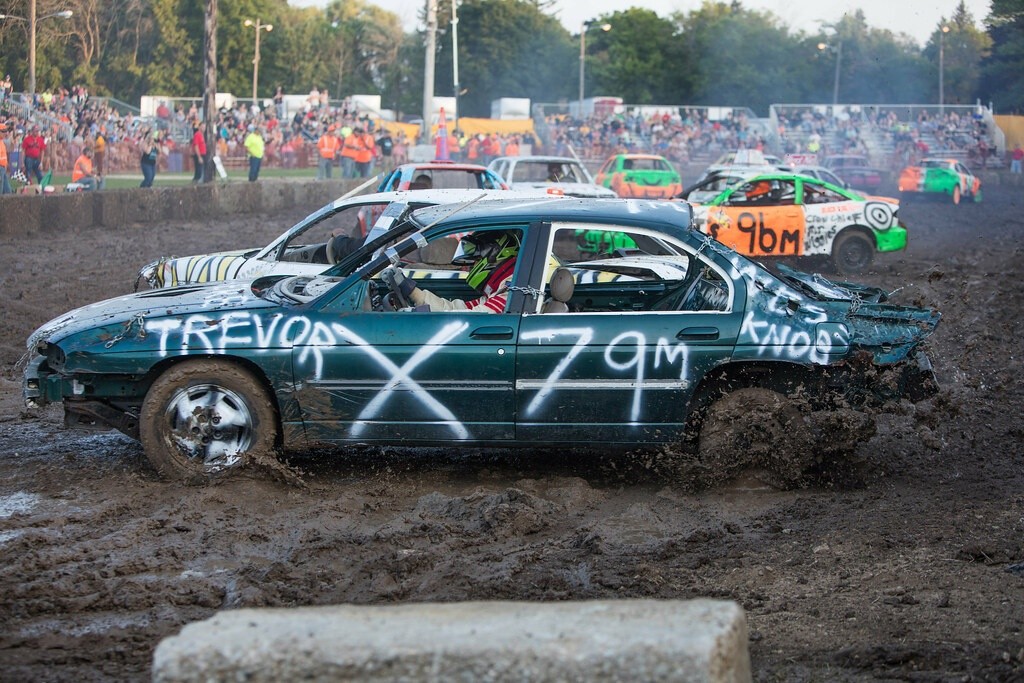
[435,107,448,160]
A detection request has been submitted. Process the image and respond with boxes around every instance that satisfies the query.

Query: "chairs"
[541,267,575,313]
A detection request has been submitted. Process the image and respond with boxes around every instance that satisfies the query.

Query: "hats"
[32,126,41,132]
[0,124,7,132]
[328,125,335,131]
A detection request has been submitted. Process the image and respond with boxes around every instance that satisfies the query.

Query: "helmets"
[463,229,523,292]
[415,175,433,189]
[746,180,772,197]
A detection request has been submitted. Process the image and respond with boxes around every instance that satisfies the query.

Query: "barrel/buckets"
[168,150,183,172]
[67,183,84,193]
[44,186,55,194]
[9,152,22,177]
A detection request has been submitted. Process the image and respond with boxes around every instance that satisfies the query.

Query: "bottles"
[21,186,28,193]
[77,186,82,191]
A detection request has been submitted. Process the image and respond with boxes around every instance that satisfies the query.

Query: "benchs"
[533,103,1006,177]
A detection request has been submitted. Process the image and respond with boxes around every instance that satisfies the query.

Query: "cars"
[661,148,909,283]
[350,160,513,245]
[897,156,984,207]
[132,187,690,293]
[487,154,622,199]
[21,195,942,492]
[594,153,684,201]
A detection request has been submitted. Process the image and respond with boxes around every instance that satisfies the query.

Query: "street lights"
[939,25,950,104]
[817,41,845,107]
[580,21,612,104]
[244,17,274,104]
[0,9,74,93]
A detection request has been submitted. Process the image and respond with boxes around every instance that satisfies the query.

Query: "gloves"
[378,265,418,300]
[332,228,346,238]
[397,304,430,313]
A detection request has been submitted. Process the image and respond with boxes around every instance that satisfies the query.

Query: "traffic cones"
[430,106,456,164]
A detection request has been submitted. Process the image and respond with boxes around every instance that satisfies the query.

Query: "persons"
[94,132,105,178]
[0,124,12,194]
[22,126,44,185]
[72,147,105,192]
[381,230,520,315]
[730,182,770,203]
[0,75,410,179]
[448,107,997,163]
[546,164,563,183]
[1010,144,1024,174]
[141,131,161,188]
